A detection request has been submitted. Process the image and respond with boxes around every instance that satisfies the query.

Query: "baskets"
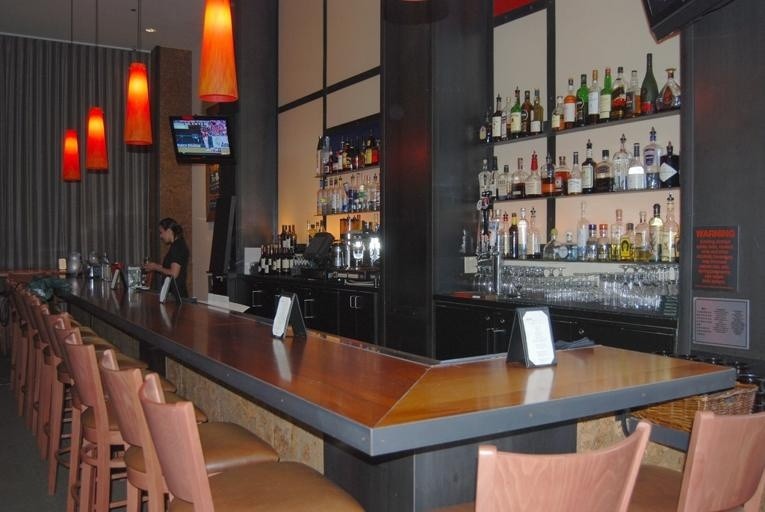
[629,383,759,433]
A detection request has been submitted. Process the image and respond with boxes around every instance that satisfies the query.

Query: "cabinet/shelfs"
[63,334,208,512]
[579,313,678,356]
[477,107,680,357]
[434,301,578,360]
[241,279,274,319]
[275,282,337,336]
[314,163,380,286]
[337,290,383,345]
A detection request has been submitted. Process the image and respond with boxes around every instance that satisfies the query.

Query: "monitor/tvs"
[168,114,236,165]
[302,232,334,267]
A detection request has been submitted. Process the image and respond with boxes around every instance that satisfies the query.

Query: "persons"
[142,216,191,301]
[200,127,213,149]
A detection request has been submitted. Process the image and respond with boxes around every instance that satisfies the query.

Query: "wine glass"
[472,264,679,311]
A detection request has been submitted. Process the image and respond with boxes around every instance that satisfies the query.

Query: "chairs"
[95,350,280,512]
[23,292,37,431]
[49,318,175,495]
[629,410,765,512]
[430,418,653,512]
[10,281,20,391]
[38,308,150,459]
[136,374,366,512]
[16,284,29,416]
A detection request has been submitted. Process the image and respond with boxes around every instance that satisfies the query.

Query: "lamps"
[198,0,238,102]
[62,0,81,181]
[86,0,108,170]
[124,0,153,145]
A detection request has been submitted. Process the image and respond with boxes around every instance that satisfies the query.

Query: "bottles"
[488,156,500,201]
[509,212,518,260]
[527,207,542,261]
[599,66,614,123]
[625,70,642,118]
[479,111,492,144]
[500,212,512,261]
[594,150,613,193]
[559,232,577,260]
[478,160,492,199]
[610,66,628,121]
[530,89,543,136]
[258,246,295,275]
[317,174,382,214]
[578,202,592,261]
[475,207,501,255]
[649,204,664,263]
[502,97,512,139]
[583,139,598,194]
[661,197,679,264]
[498,166,514,202]
[628,143,646,192]
[661,141,680,189]
[641,53,660,115]
[611,208,627,261]
[517,208,528,262]
[620,224,634,262]
[634,212,649,263]
[510,88,524,138]
[588,70,602,126]
[552,96,565,132]
[280,224,297,250]
[657,68,681,112]
[554,156,571,196]
[320,133,382,174]
[577,74,592,128]
[543,228,563,260]
[512,156,527,199]
[540,154,556,196]
[522,91,533,137]
[564,79,577,129]
[645,128,661,190]
[308,219,325,241]
[568,152,583,195]
[525,150,542,198]
[492,95,504,141]
[598,224,611,262]
[586,225,599,262]
[613,134,630,191]
